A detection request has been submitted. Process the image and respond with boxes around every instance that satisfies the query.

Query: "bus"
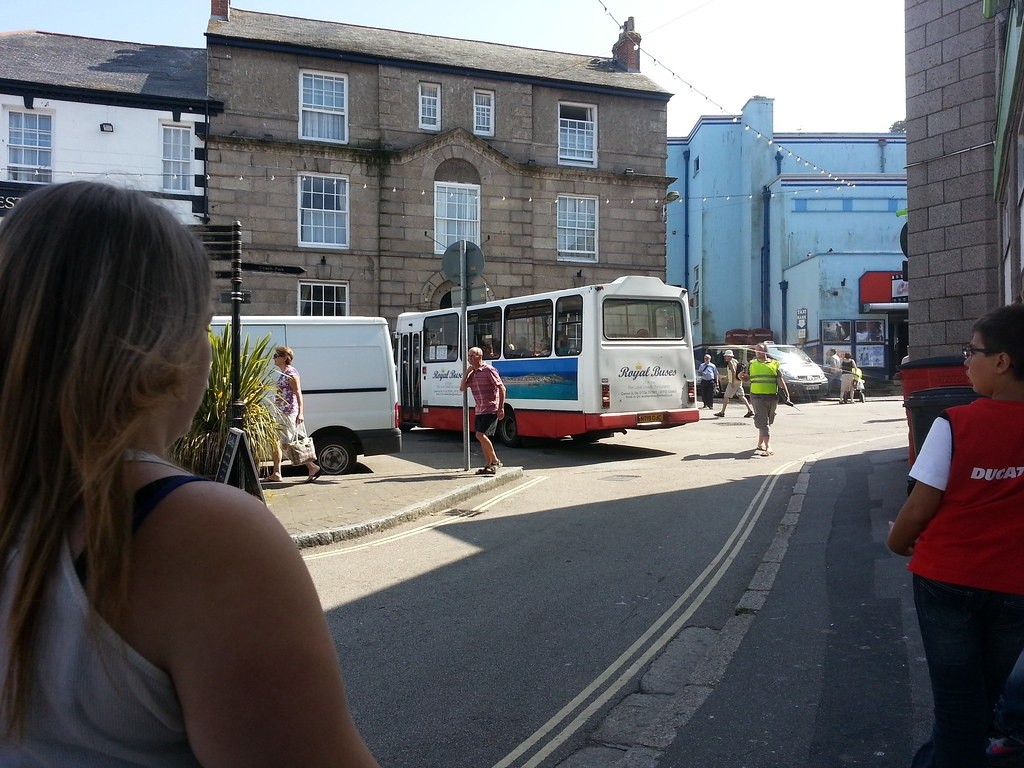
[392,276,701,448]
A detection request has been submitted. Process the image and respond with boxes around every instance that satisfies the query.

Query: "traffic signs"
[181,221,306,303]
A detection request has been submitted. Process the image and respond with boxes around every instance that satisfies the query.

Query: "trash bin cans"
[898,356,991,499]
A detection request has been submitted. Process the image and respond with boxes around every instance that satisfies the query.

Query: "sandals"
[475,459,503,475]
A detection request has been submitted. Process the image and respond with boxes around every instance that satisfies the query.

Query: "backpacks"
[735,363,747,380]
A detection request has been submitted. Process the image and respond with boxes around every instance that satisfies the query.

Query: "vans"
[706,345,830,403]
[207,316,401,475]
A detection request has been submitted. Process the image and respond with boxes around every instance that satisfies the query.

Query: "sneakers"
[760,447,774,456]
[758,444,765,451]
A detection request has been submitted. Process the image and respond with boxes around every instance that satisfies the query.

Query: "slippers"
[260,476,282,483]
[305,468,322,482]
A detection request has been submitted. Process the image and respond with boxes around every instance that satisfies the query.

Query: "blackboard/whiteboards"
[212,427,242,484]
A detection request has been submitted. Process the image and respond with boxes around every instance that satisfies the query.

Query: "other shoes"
[744,411,754,417]
[824,393,830,397]
[709,406,713,409]
[850,401,854,403]
[703,405,707,408]
[714,412,724,417]
[840,400,843,403]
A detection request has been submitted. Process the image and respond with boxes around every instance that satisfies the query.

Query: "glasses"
[962,341,998,357]
[273,354,285,358]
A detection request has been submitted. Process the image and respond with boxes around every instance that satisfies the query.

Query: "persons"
[836,323,843,339]
[713,350,754,417]
[737,344,790,455]
[484,332,573,359]
[0,181,381,768]
[260,346,320,483]
[839,352,857,404]
[824,349,841,396]
[460,346,506,475]
[698,354,719,409]
[887,305,1023,768]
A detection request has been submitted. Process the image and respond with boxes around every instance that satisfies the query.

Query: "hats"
[704,354,711,359]
[723,349,734,356]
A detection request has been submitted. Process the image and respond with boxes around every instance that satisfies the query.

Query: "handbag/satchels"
[287,421,318,466]
